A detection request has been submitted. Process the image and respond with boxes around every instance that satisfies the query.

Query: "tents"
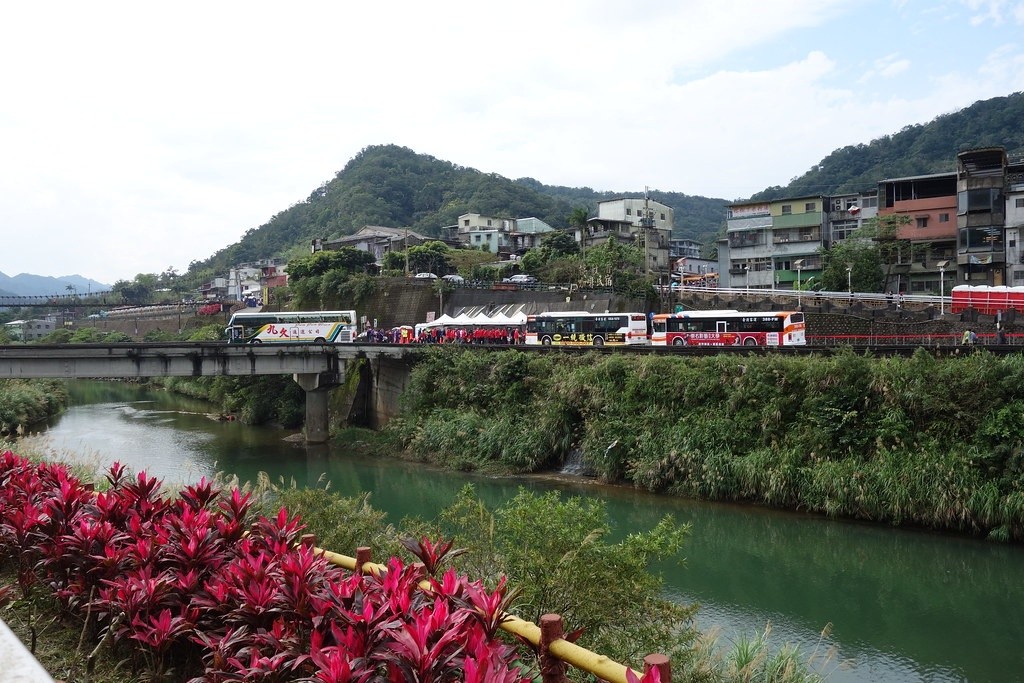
[427,310,527,327]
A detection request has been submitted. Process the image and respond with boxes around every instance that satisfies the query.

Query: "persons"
[900,292,904,302]
[962,327,978,345]
[885,291,893,303]
[367,327,519,345]
[671,279,715,292]
[997,326,1007,343]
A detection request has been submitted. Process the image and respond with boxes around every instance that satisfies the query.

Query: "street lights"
[794,259,804,308]
[702,264,708,291]
[936,260,950,315]
[744,266,749,298]
[678,265,685,300]
[845,268,852,301]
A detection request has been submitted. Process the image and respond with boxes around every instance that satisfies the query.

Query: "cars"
[415,273,436,279]
[502,275,537,284]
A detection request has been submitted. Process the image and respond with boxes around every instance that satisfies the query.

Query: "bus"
[225,310,358,347]
[649,309,807,347]
[524,311,647,347]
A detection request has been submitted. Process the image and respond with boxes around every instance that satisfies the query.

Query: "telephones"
[248,296,256,307]
